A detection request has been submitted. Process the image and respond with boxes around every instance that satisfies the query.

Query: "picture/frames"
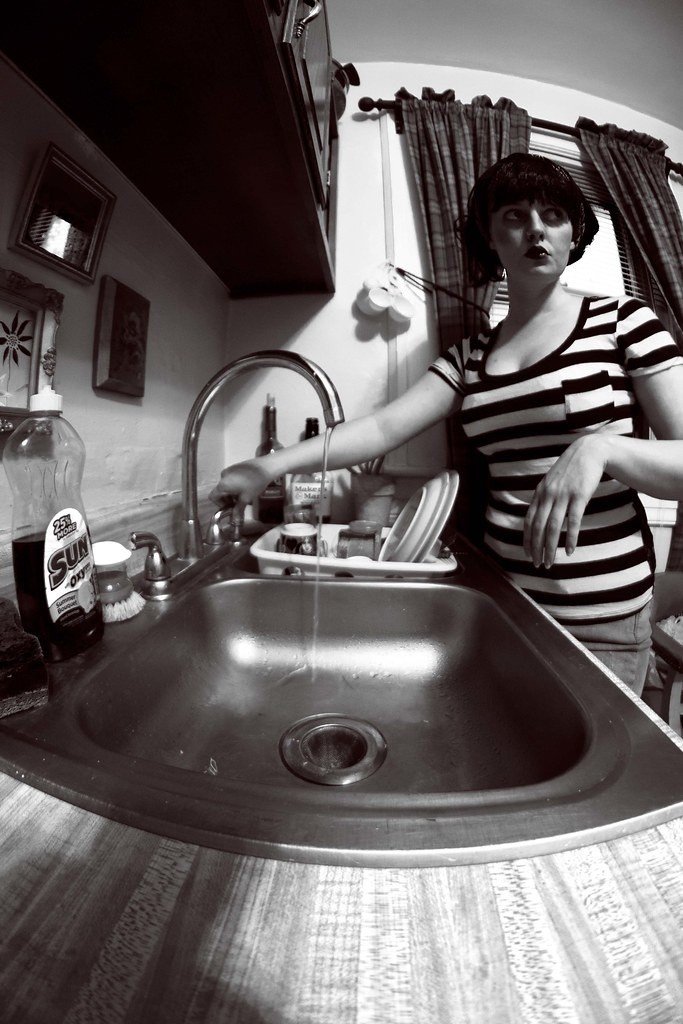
[6,135,117,287]
[91,275,151,398]
[0,267,67,435]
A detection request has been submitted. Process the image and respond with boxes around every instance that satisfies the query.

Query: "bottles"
[290,417,334,523]
[253,392,285,523]
[3,385,104,660]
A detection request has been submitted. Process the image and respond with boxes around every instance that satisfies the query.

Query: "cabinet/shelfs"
[0,0,339,299]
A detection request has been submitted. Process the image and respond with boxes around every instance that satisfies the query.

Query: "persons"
[207,151,683,699]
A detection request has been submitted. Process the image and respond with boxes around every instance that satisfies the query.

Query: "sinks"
[0,575,682,866]
[205,525,502,577]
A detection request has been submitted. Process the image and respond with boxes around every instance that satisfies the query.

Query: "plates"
[378,469,459,563]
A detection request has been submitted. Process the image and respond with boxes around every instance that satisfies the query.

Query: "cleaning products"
[0,382,104,667]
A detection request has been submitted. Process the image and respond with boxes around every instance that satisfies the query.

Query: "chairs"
[641,568,683,739]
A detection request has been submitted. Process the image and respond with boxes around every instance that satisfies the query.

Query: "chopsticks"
[345,456,385,473]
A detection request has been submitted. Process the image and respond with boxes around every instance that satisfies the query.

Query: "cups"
[279,522,318,556]
[350,520,383,561]
[337,531,375,560]
[351,474,396,527]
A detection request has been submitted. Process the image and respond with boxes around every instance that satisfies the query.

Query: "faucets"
[177,349,344,562]
